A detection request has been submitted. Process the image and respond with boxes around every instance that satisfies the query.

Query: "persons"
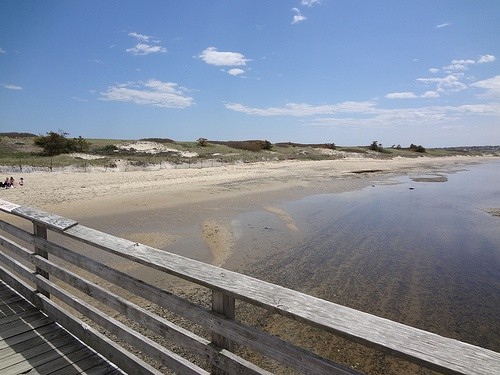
[19,177,24,187]
[9,176,16,185]
[4,177,11,188]
